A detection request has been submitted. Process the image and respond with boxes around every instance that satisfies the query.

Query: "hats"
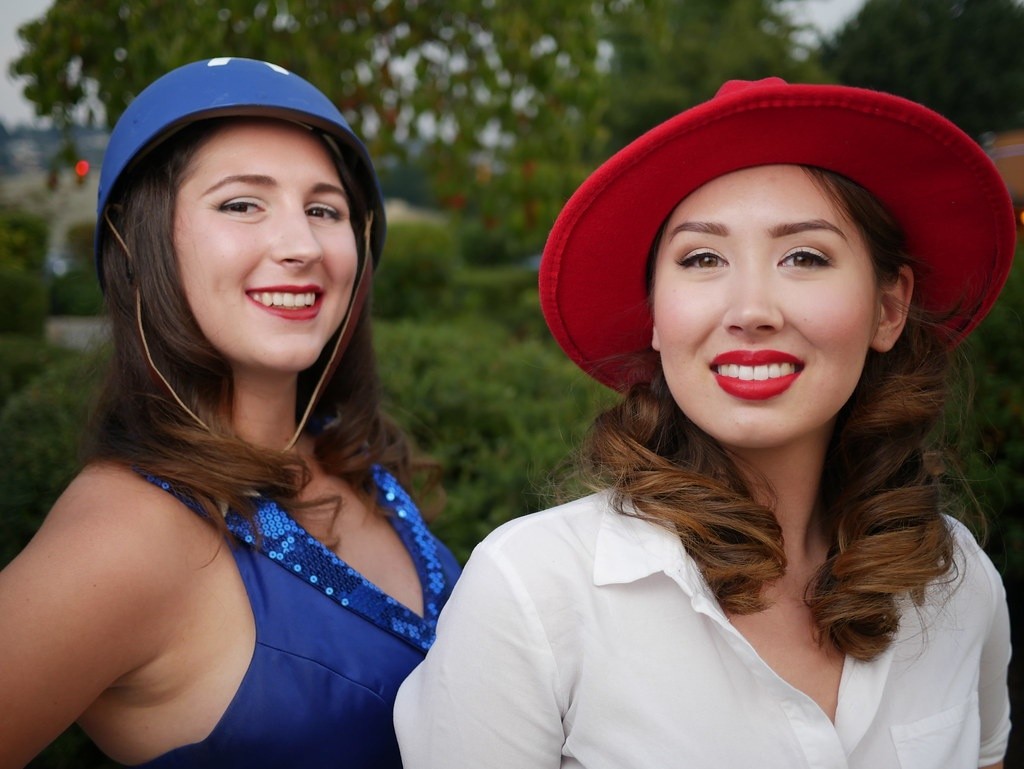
[538,78,1017,400]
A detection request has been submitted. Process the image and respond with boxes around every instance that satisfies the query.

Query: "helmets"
[94,56,387,304]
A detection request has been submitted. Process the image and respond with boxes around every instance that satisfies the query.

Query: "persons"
[392,77,1016,768]
[0,57,461,769]
